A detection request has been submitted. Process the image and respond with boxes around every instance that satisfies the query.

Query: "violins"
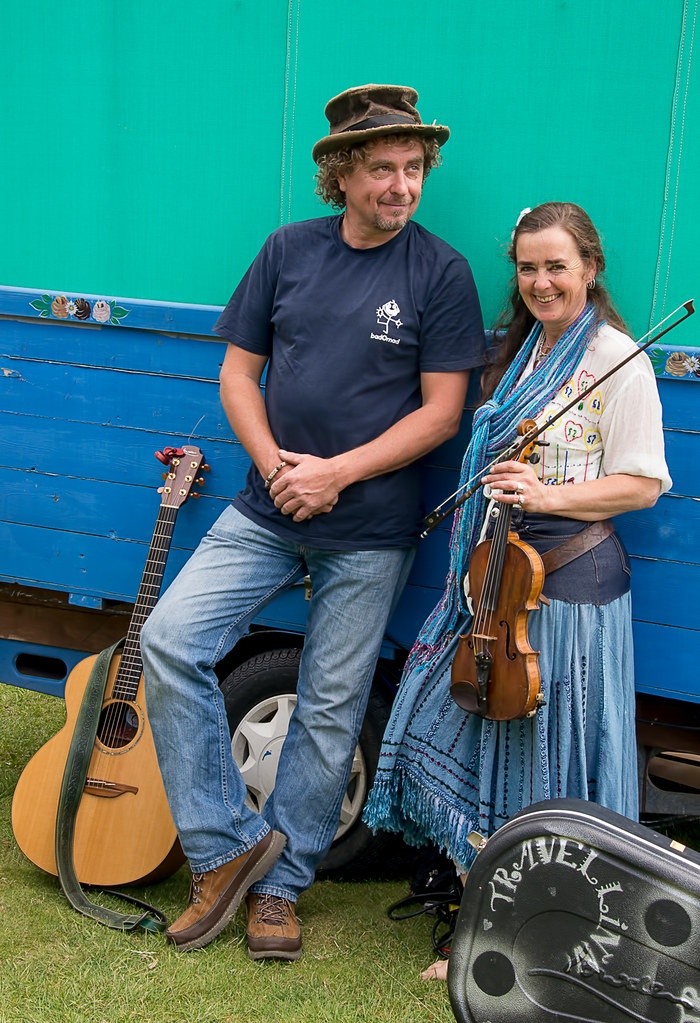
[450,417,547,722]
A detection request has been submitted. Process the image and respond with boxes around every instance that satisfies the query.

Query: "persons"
[360,203,673,981]
[141,83,488,958]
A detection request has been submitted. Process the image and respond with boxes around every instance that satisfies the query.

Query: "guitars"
[11,451,188,888]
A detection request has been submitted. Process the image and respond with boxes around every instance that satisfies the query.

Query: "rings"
[518,494,524,504]
[516,482,523,493]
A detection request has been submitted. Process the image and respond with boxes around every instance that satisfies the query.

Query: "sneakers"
[240,888,304,962]
[164,828,287,951]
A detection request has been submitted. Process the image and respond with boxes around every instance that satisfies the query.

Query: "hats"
[311,83,451,168]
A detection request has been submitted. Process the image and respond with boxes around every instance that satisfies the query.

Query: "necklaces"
[536,332,550,369]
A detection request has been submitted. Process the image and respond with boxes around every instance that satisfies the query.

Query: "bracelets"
[264,462,289,488]
